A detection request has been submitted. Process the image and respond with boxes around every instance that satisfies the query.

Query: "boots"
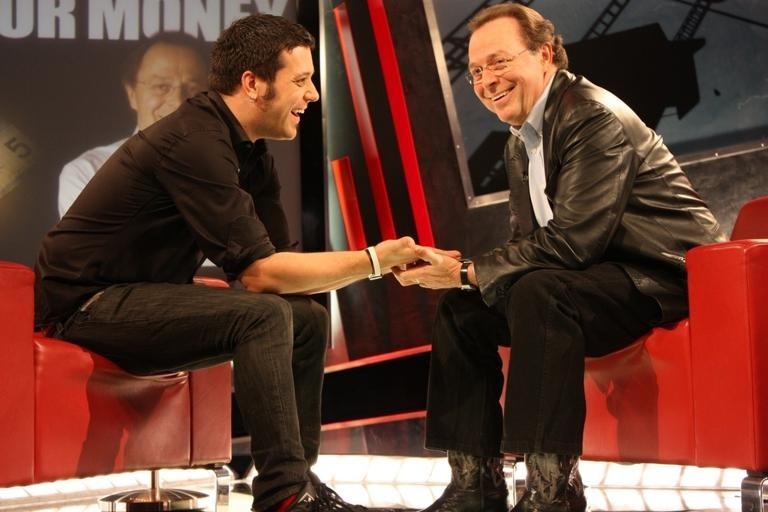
[421,450,586,512]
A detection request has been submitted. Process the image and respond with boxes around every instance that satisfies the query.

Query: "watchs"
[362,245,384,282]
[459,261,478,292]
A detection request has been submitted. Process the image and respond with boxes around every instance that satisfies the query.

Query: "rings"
[415,279,419,284]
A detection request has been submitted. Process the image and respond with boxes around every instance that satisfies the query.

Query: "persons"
[384,2,732,512]
[57,28,210,223]
[30,11,466,512]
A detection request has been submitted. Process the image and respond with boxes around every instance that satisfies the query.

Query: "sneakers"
[284,479,366,512]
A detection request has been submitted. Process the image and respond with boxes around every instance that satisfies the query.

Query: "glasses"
[133,78,201,98]
[463,48,529,84]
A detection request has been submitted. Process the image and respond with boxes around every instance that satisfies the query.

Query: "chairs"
[574,193,768,512]
[0,260,233,512]
